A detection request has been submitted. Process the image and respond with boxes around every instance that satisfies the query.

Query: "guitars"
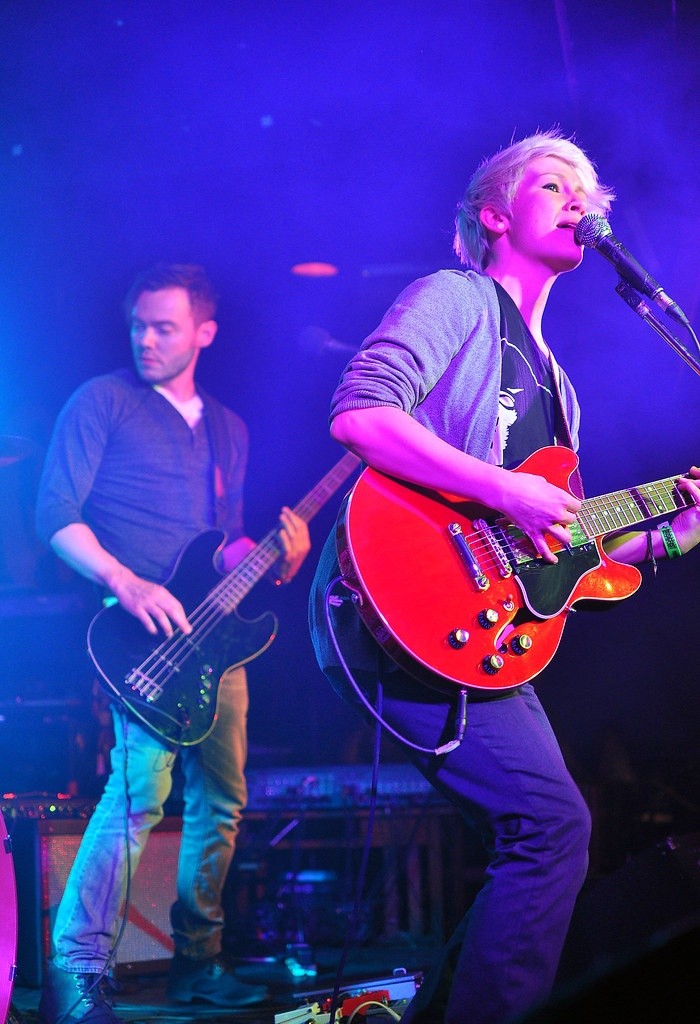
[84,449,359,750]
[333,445,700,703]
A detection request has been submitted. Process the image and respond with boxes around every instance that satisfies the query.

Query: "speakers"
[12,814,185,987]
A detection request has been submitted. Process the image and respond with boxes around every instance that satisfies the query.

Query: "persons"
[35,267,312,1023]
[308,134,700,1023]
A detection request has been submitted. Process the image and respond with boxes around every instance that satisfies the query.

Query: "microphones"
[573,213,690,330]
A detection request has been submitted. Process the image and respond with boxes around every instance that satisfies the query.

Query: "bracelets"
[657,520,682,560]
[646,529,659,577]
